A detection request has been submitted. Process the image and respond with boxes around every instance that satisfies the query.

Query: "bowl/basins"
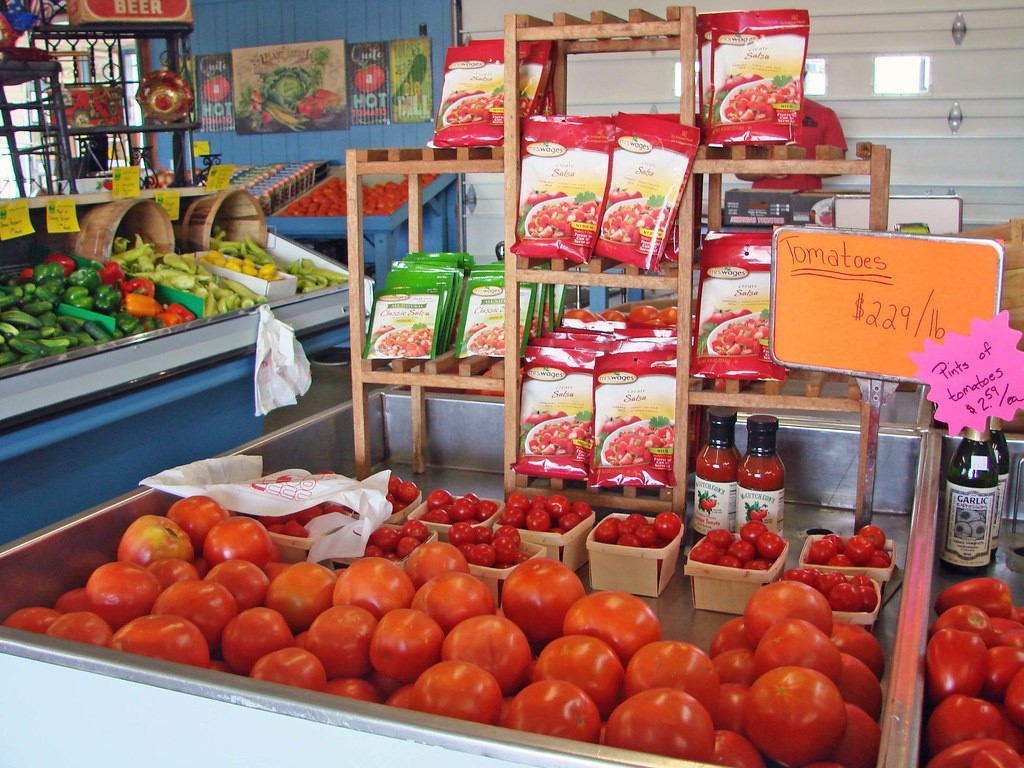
[75,178,115,194]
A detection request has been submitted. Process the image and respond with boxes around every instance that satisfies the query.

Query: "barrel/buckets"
[179,188,268,253]
[65,198,177,265]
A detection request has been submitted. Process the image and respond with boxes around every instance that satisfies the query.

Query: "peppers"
[21,223,350,359]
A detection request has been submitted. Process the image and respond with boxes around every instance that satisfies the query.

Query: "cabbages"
[257,67,313,113]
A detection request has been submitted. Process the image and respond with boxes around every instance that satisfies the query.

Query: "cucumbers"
[0,274,114,367]
[391,54,427,106]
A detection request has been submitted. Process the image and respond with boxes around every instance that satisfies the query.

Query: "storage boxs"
[46,86,125,128]
[67,0,194,26]
[468,540,547,609]
[407,496,505,545]
[187,250,297,302]
[585,513,685,598]
[269,528,338,564]
[329,522,439,568]
[345,489,423,525]
[799,534,897,590]
[492,500,595,572]
[788,190,870,227]
[723,189,801,228]
[826,573,882,632]
[683,534,789,616]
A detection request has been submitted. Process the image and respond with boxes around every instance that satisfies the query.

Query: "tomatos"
[369,324,432,359]
[466,305,557,357]
[1,472,891,768]
[521,188,669,245]
[564,303,681,328]
[280,173,440,215]
[704,309,771,355]
[705,72,802,123]
[523,411,676,467]
[920,576,1024,768]
[439,89,532,127]
[354,64,386,92]
[204,71,230,102]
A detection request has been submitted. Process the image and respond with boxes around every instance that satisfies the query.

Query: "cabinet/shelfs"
[344,6,892,531]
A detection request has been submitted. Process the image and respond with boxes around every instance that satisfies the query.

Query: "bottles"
[692,407,741,539]
[938,416,999,578]
[988,415,1011,557]
[736,415,787,539]
